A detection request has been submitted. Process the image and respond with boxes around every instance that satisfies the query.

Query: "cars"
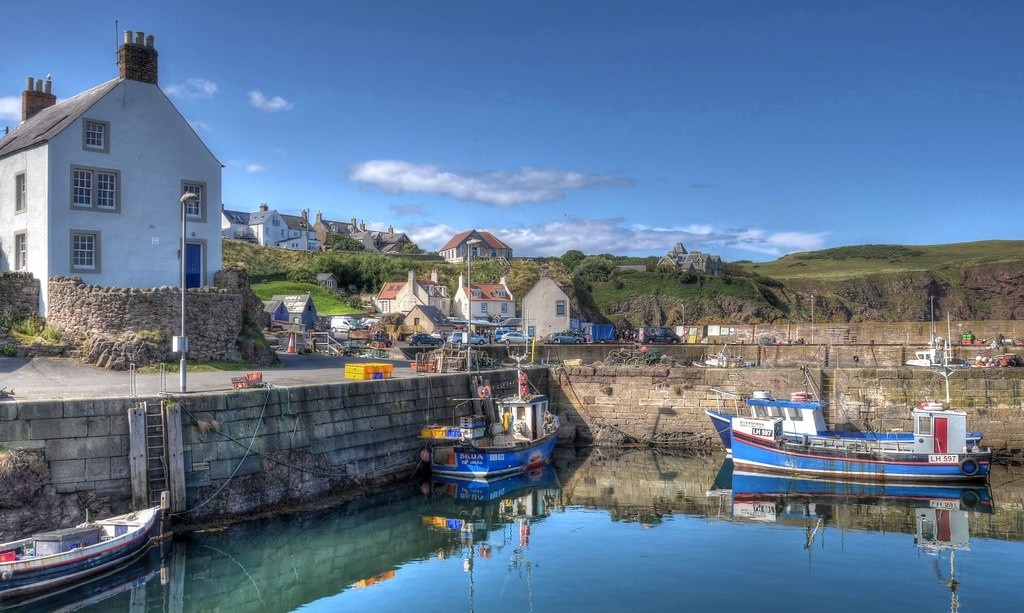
[498,332,533,344]
[373,335,392,347]
[405,333,444,347]
[547,332,585,345]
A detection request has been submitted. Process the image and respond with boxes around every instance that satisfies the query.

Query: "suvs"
[447,332,487,345]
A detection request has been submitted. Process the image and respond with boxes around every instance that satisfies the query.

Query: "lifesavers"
[791,391,813,402]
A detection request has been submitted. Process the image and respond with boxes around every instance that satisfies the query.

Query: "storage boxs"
[422,415,486,439]
[346,364,392,379]
[410,362,434,371]
[32,528,105,555]
[962,334,988,345]
[565,359,581,366]
[233,373,261,388]
[98,522,128,537]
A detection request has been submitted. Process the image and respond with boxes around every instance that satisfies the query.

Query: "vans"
[330,316,363,333]
[638,326,681,345]
[494,329,517,344]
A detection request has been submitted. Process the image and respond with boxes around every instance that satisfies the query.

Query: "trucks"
[582,324,615,344]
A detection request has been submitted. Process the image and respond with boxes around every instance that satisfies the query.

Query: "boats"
[418,343,561,478]
[418,464,563,573]
[703,310,992,486]
[707,454,998,613]
[691,343,756,368]
[0,551,171,612]
[905,294,969,369]
[0,490,175,612]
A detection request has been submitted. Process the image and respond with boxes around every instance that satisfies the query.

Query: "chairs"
[490,415,514,445]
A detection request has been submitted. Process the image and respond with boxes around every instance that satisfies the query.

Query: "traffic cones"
[287,333,297,353]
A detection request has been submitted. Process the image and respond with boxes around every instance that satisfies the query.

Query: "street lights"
[169,192,199,395]
[681,304,686,344]
[465,239,484,373]
[810,295,814,344]
